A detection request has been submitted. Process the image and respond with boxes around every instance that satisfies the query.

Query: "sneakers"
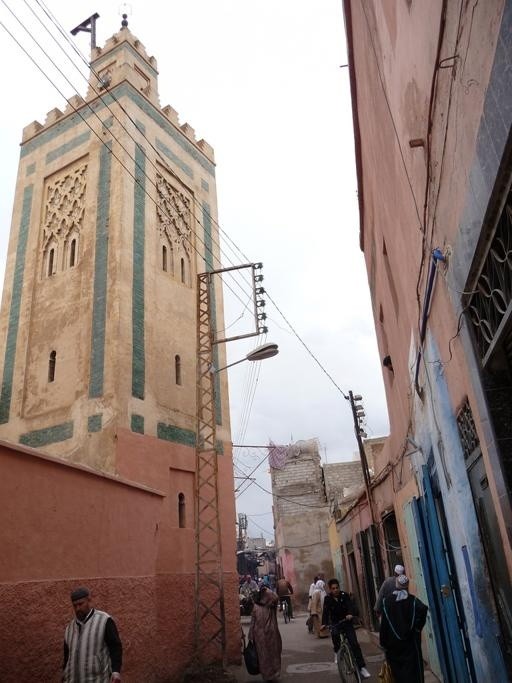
[360,667,371,678]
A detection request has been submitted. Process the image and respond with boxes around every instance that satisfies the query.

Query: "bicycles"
[323,616,364,683]
[279,597,295,624]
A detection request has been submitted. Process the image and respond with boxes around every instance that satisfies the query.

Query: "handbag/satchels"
[334,652,338,664]
[319,629,331,636]
[376,659,396,683]
[244,638,260,675]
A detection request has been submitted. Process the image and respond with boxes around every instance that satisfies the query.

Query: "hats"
[396,575,409,589]
[395,564,404,575]
[70,586,89,601]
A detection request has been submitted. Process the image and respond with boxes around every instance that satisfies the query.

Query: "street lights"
[196,342,279,674]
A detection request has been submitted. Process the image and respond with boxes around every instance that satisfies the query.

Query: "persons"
[62,587,124,682]
[236,570,276,594]
[319,578,372,678]
[373,564,408,620]
[276,575,295,619]
[249,584,283,683]
[307,576,319,614]
[381,574,429,682]
[311,579,332,639]
